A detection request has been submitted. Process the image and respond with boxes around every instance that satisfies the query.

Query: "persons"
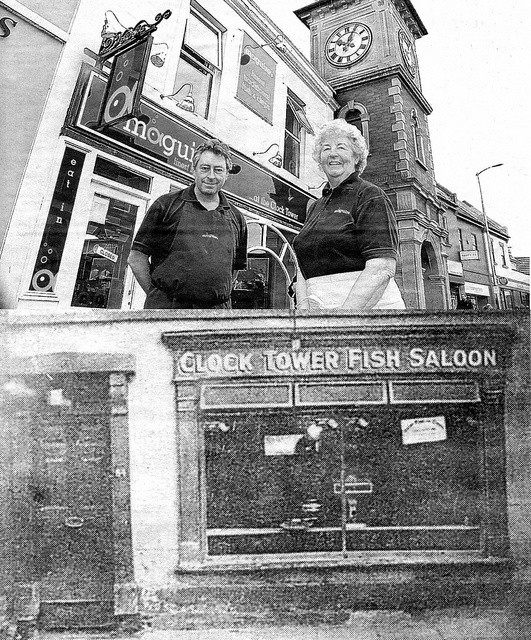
[127,140,246,309]
[293,118,405,308]
[456,293,473,310]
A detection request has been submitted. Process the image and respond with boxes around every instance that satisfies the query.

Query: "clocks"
[324,21,373,69]
[398,27,419,78]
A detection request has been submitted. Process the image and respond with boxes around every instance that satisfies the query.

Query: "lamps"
[244,33,288,55]
[103,10,170,68]
[252,142,284,168]
[160,81,197,114]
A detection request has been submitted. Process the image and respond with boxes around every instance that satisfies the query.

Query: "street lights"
[465,151,521,321]
